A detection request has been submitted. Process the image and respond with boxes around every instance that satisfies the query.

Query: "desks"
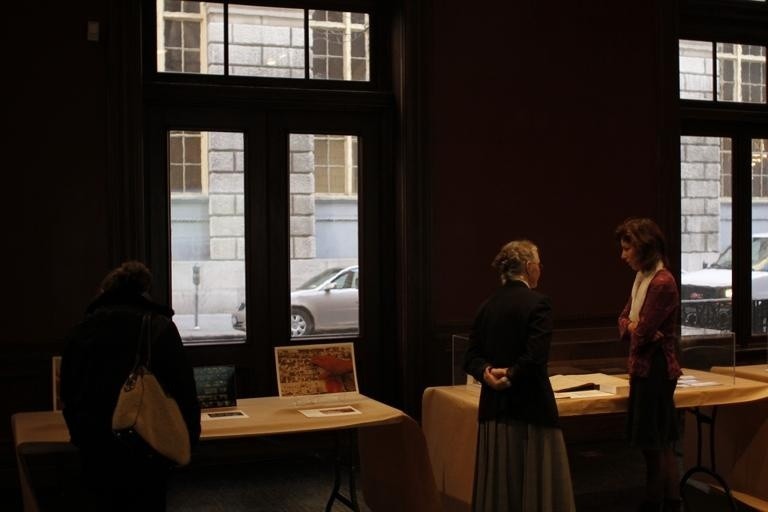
[422,360,768,508]
[10,394,405,511]
[345,364,429,510]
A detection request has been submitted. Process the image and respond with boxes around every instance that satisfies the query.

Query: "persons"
[613,216,685,511]
[460,239,576,512]
[54,259,202,512]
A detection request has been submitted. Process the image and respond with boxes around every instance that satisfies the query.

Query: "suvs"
[681,233,768,300]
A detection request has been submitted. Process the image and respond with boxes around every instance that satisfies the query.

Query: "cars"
[231,265,359,337]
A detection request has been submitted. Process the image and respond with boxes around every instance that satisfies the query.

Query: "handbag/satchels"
[113,369,192,469]
